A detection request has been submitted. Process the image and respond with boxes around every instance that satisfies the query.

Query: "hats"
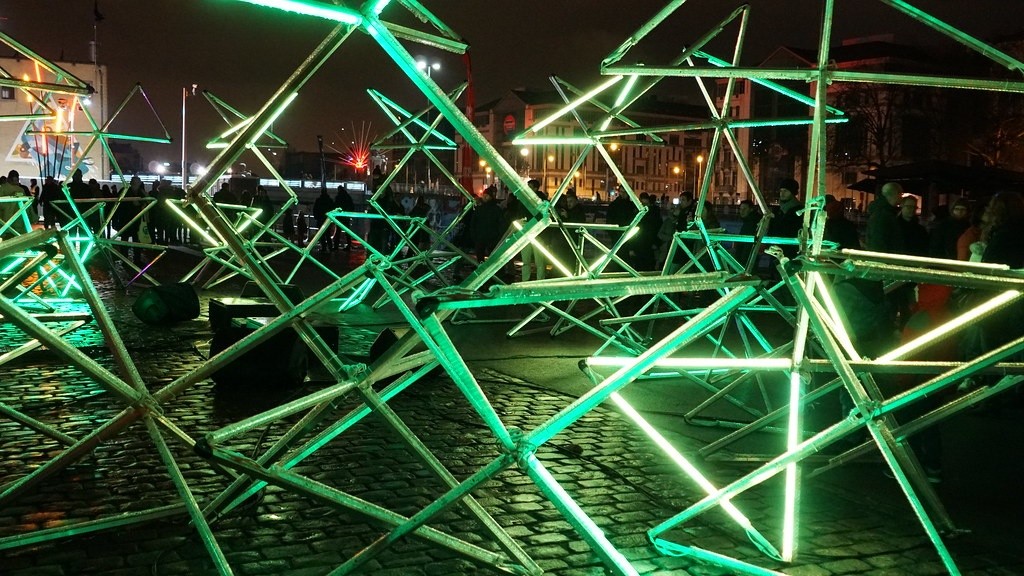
[779,178,799,195]
[483,188,493,197]
[8,170,19,177]
[372,165,381,175]
[953,198,970,209]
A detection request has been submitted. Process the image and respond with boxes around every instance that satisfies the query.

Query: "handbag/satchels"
[657,218,673,242]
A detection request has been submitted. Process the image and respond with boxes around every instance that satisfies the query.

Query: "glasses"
[954,208,968,212]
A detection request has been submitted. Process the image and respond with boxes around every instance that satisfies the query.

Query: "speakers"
[132,283,201,327]
[371,326,404,365]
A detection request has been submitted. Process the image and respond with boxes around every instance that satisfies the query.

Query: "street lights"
[417,61,440,190]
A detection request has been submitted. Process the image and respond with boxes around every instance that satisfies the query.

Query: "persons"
[606,184,721,297]
[459,178,585,286]
[281,181,354,253]
[144,178,274,251]
[366,184,436,273]
[731,177,862,315]
[0,168,145,261]
[828,181,1023,484]
